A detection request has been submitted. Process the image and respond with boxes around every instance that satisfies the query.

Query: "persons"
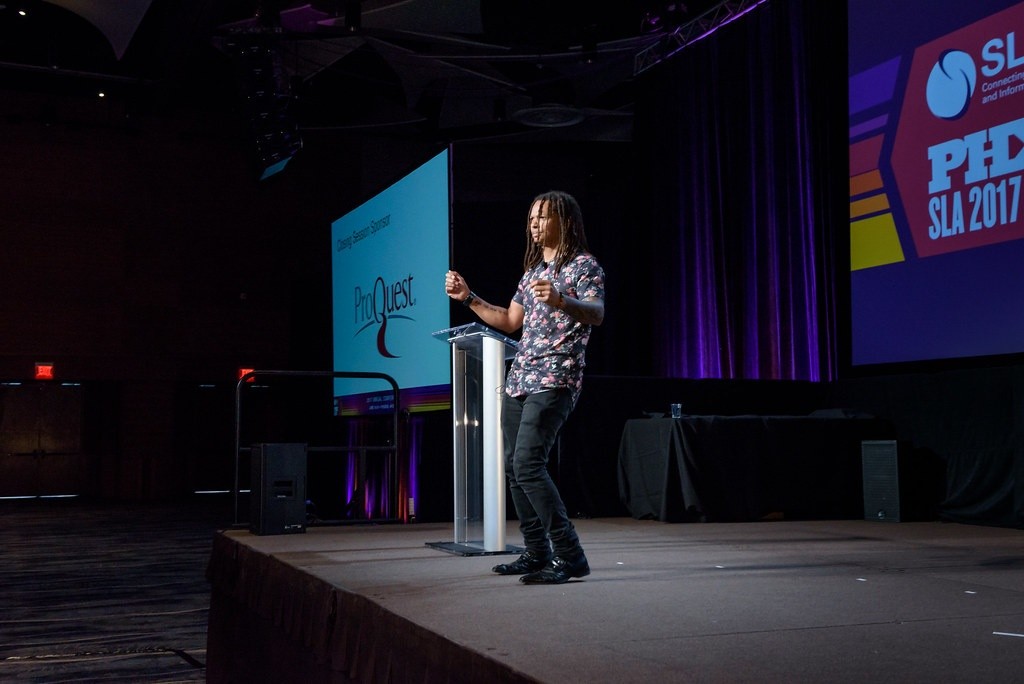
[445,190,604,586]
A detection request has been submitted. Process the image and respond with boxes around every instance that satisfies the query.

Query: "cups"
[671,403,683,419]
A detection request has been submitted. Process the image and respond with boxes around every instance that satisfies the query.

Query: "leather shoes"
[492,551,553,574]
[519,553,590,585]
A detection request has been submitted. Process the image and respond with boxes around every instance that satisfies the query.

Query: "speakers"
[862,438,936,522]
[250,443,307,535]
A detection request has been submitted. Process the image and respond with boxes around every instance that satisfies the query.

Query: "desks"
[615,413,886,523]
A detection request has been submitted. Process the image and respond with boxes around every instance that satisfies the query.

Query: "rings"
[540,291,542,296]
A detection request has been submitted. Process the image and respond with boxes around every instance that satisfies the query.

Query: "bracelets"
[463,291,476,306]
[556,292,566,309]
